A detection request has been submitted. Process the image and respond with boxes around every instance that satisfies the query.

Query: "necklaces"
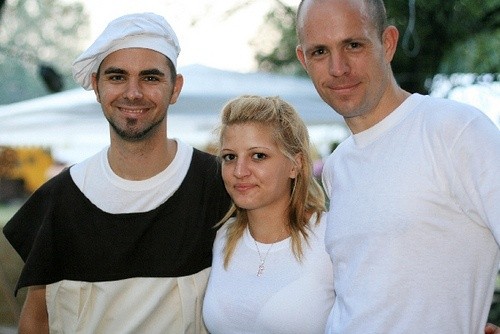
[251,221,289,278]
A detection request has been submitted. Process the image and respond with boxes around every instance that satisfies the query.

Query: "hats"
[73,12,180,91]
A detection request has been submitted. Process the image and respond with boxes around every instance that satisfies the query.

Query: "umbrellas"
[1,61,343,153]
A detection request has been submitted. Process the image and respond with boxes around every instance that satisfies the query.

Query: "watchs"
[486,321,497,334]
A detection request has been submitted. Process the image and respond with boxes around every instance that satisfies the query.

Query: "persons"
[0,12,234,334]
[203,94,500,334]
[292,0,500,334]
